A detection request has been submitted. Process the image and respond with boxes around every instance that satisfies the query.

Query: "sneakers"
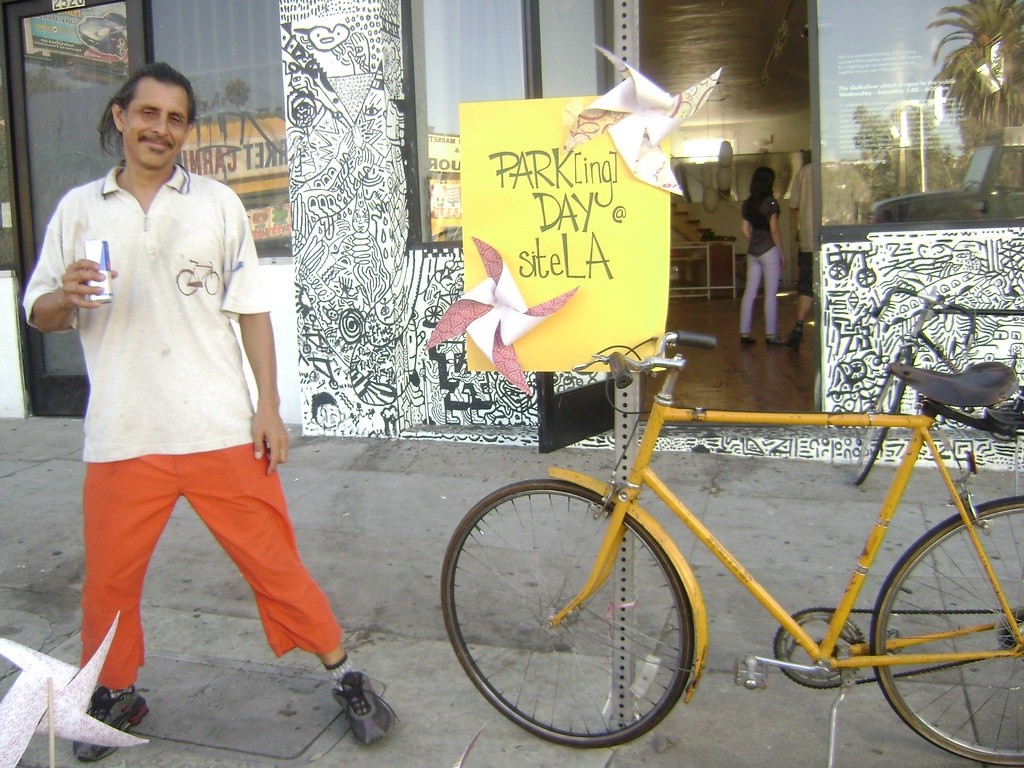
[331,670,400,746]
[73,682,150,762]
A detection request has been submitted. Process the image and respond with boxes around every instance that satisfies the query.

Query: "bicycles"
[439,326,1024,768]
[854,284,1024,486]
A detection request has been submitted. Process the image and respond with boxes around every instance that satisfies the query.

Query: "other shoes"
[740,335,756,346]
[787,321,804,348]
[766,336,786,345]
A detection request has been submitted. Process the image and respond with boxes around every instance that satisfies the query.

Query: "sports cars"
[78,11,128,59]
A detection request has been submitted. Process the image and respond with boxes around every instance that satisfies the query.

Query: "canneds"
[83,238,112,303]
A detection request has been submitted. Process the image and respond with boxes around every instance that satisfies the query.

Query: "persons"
[738,167,789,344]
[788,138,870,346]
[852,105,894,195]
[23,62,395,764]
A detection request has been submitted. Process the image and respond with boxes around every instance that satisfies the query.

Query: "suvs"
[867,144,1024,224]
